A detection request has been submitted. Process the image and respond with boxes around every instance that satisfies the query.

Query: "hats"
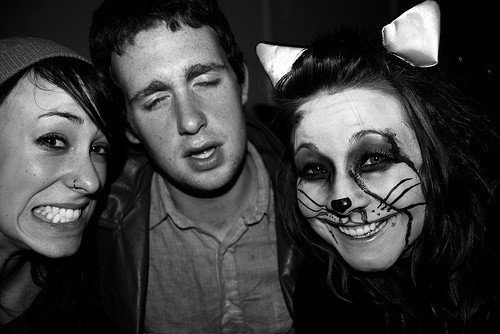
[0,36,102,88]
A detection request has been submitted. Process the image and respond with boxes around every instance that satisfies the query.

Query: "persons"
[89,0,299,334]
[256,0,500,334]
[0,38,120,334]
